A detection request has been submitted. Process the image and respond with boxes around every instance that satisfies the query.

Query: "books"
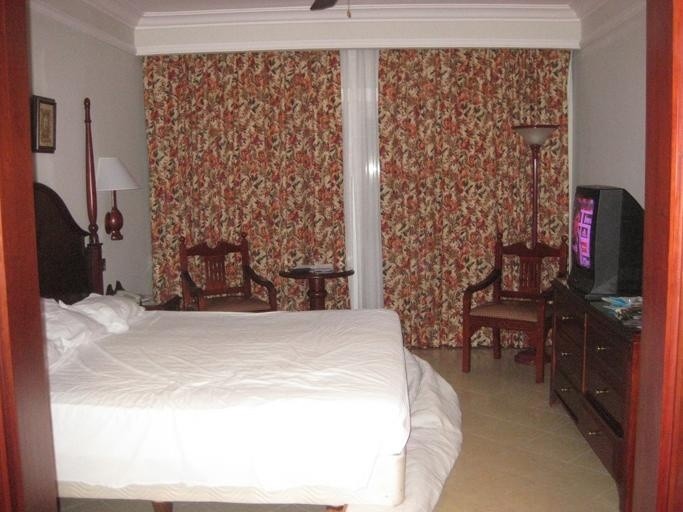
[291,263,333,274]
[600,295,642,329]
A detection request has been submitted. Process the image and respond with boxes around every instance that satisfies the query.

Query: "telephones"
[114,290,142,306]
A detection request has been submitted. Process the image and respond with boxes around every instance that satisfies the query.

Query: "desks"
[280,263,355,312]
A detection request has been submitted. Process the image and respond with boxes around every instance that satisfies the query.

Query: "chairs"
[179,231,276,314]
[462,233,568,385]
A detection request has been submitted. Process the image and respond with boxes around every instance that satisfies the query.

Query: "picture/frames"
[34,95,58,155]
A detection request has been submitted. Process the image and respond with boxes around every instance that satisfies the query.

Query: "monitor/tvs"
[567,182,644,300]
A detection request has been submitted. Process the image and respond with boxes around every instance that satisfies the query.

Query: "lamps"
[92,156,140,242]
[514,124,552,366]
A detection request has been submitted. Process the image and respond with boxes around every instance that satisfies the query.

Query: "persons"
[571,201,581,265]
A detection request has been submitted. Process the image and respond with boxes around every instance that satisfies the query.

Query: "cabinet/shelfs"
[548,278,643,512]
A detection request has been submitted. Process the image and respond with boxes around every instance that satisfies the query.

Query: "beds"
[32,98,411,512]
